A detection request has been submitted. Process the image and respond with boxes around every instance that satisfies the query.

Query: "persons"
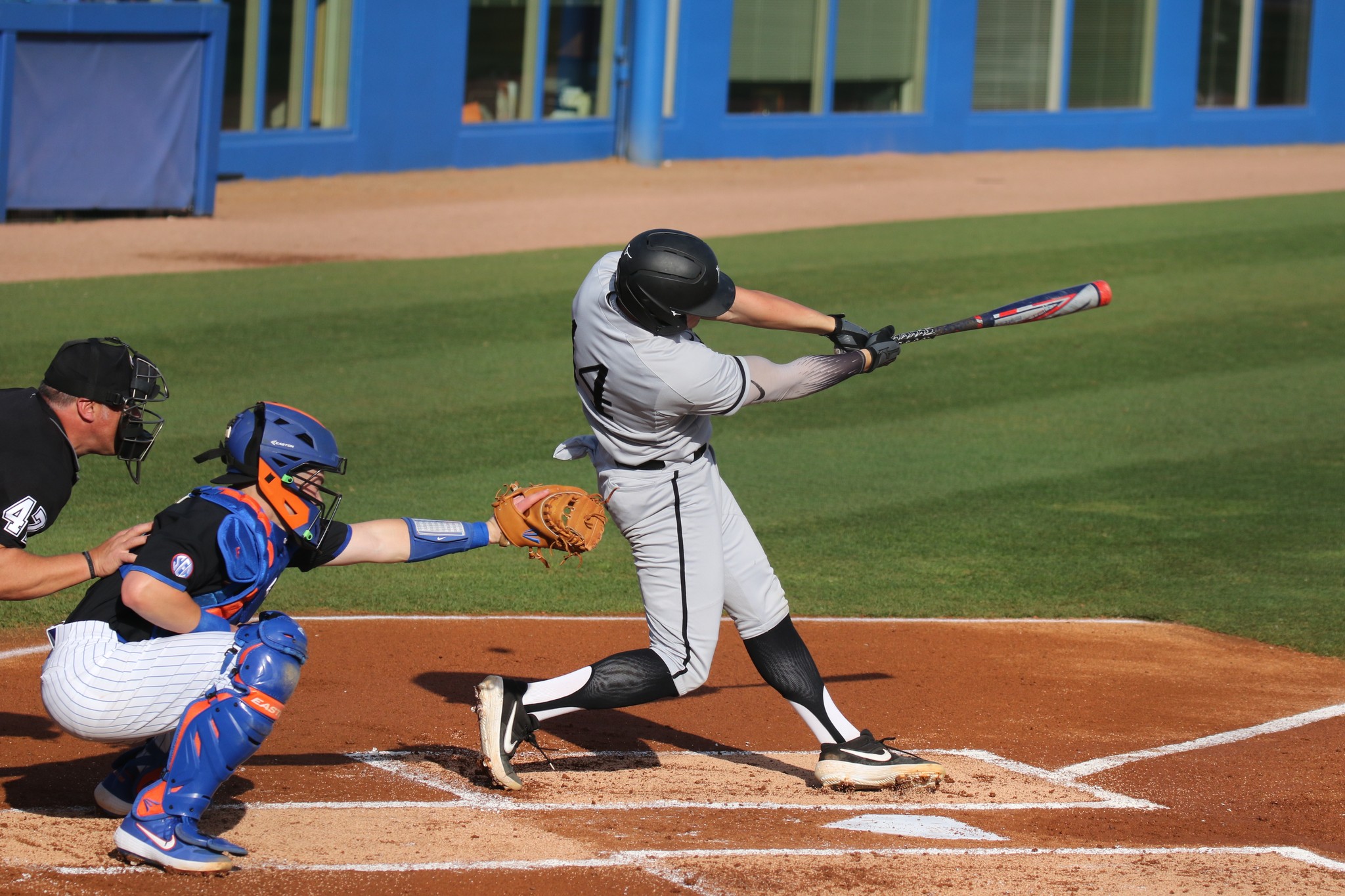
[39,401,609,874]
[0,336,170,601]
[478,230,948,788]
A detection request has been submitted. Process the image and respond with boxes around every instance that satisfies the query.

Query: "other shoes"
[463,107,494,130]
[548,87,593,121]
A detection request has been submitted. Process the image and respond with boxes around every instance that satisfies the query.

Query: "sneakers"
[114,811,235,876]
[474,674,567,792]
[95,766,141,818]
[815,729,945,791]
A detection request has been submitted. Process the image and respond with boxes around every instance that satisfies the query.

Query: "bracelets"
[82,551,97,579]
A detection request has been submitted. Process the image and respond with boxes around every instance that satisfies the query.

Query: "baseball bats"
[833,279,1113,356]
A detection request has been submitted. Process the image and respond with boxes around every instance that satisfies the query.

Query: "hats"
[46,338,139,412]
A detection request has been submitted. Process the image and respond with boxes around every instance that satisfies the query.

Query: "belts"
[617,444,707,470]
[47,628,126,644]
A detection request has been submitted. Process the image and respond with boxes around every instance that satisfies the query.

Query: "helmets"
[615,228,736,338]
[192,400,348,551]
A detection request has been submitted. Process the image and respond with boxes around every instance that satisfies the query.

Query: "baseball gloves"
[493,483,607,554]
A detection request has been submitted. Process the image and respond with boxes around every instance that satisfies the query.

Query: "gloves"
[864,325,900,373]
[819,314,871,353]
